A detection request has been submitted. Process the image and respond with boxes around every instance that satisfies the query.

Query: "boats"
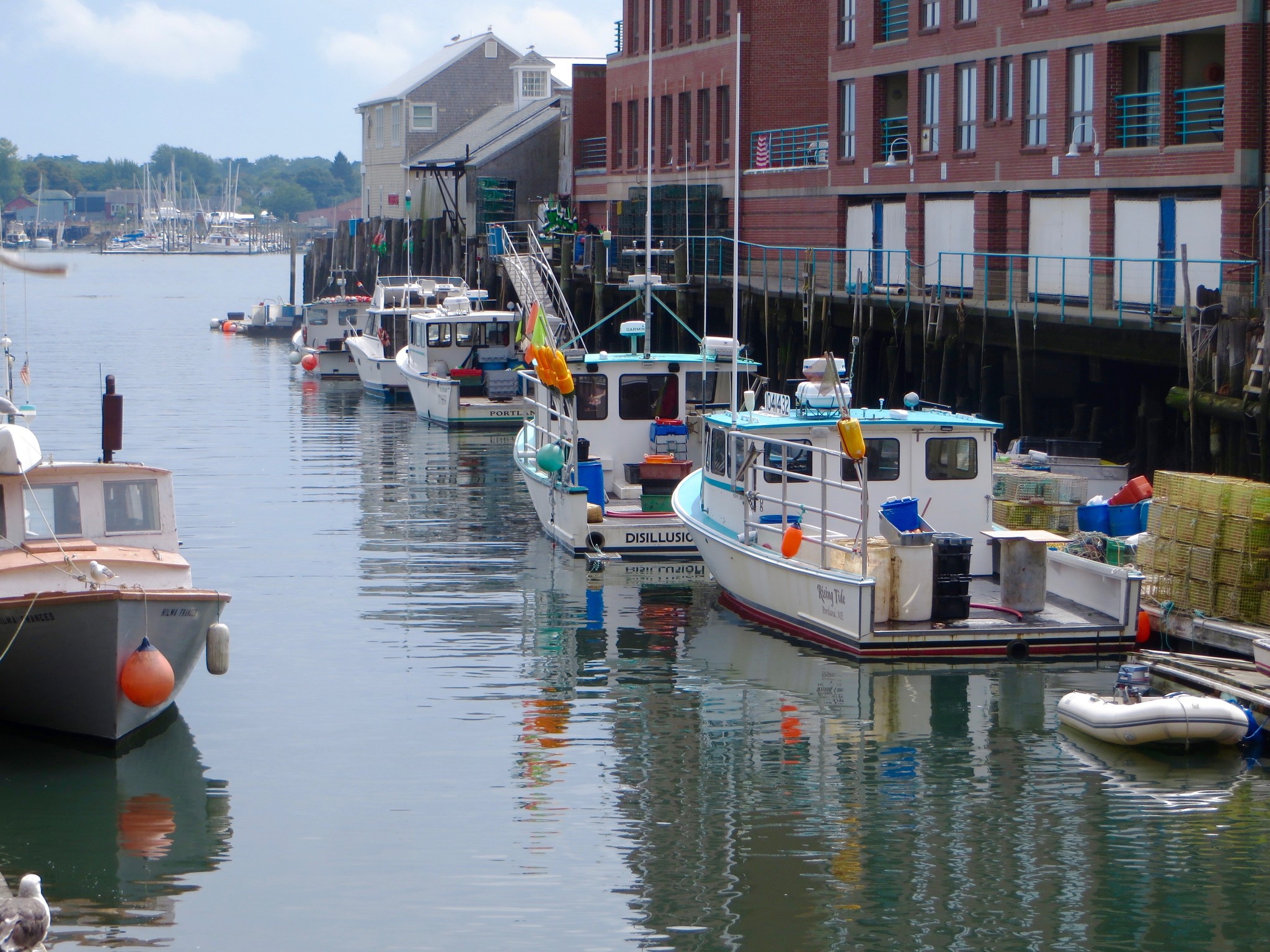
[290,197,374,378]
[344,150,498,403]
[1253,638,1270,679]
[1053,665,1249,750]
[395,260,567,431]
[0,228,231,747]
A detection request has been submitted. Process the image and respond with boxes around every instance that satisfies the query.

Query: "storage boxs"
[612,417,694,512]
[450,348,518,398]
[878,509,974,618]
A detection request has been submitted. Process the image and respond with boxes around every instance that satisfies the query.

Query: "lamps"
[1065,123,1099,156]
[885,138,913,166]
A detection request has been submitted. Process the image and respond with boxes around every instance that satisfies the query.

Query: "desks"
[797,147,820,165]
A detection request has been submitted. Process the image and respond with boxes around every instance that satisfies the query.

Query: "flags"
[515,299,548,365]
[19,357,31,385]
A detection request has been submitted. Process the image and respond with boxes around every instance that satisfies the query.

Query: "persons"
[574,219,602,264]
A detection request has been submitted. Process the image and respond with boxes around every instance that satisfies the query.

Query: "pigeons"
[89,561,120,590]
[0,396,26,418]
[0,874,51,952]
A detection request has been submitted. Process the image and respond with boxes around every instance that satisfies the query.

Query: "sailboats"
[0,148,290,258]
[667,12,1153,665]
[512,0,796,565]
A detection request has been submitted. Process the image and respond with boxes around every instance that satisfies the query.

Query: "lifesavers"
[302,326,307,343]
[377,328,391,347]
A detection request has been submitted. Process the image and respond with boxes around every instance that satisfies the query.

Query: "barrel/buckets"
[489,226,508,255]
[478,361,529,395]
[1077,498,1151,537]
[349,219,360,236]
[267,304,296,324]
[575,234,585,264]
[759,515,802,524]
[655,416,683,425]
[557,459,605,516]
[252,305,266,325]
[644,453,674,463]
[428,360,450,379]
[830,537,934,623]
[1000,538,1047,612]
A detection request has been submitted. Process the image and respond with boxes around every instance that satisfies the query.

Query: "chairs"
[806,141,828,165]
